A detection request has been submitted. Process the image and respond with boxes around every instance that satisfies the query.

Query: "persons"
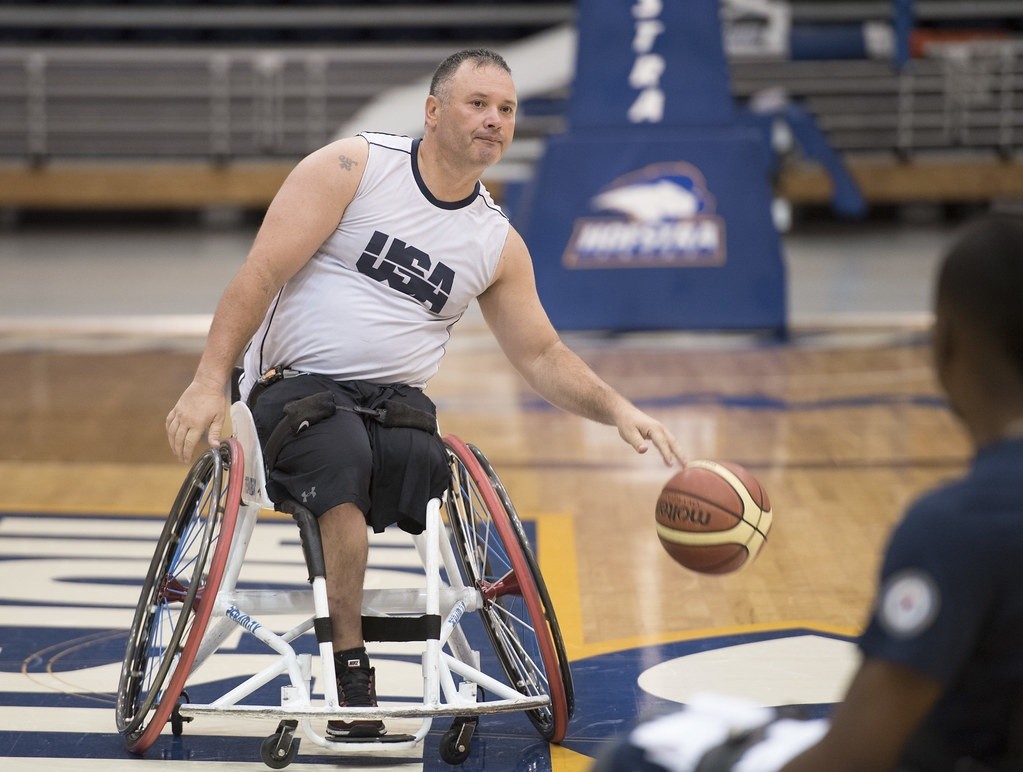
[776,215,1023,772]
[169,47,687,737]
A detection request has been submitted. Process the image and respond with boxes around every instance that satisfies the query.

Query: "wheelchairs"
[115,338,574,770]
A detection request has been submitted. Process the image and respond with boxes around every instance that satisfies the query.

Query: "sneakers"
[325,651,388,735]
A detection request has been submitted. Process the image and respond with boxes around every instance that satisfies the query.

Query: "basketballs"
[652,458,774,576]
